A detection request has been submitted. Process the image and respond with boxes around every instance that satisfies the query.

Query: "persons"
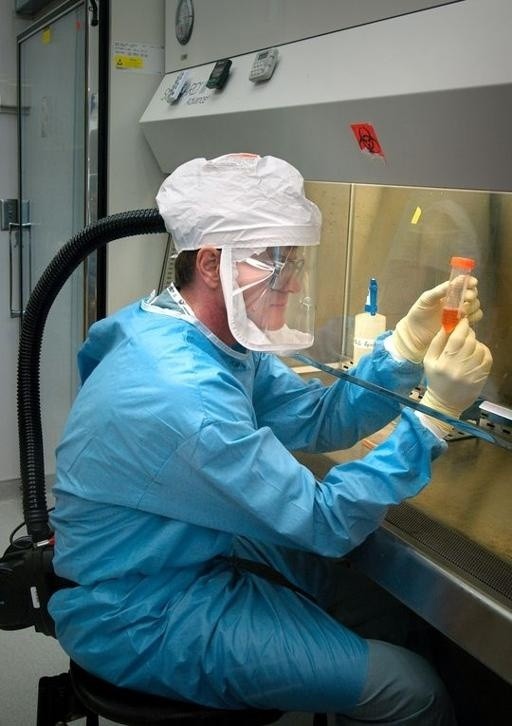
[47,152,494,724]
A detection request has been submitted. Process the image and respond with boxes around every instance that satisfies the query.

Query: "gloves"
[392,275,493,438]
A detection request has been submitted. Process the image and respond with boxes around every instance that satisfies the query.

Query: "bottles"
[443,258,472,334]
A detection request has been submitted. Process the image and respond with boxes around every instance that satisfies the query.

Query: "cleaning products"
[354,279,386,372]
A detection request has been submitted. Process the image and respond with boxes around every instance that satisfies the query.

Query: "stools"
[33,662,329,726]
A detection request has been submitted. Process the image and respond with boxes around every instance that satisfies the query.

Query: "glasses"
[245,257,306,290]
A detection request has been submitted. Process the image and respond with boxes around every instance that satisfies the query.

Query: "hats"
[155,153,322,254]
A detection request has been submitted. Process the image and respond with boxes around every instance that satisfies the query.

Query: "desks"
[262,360,511,683]
